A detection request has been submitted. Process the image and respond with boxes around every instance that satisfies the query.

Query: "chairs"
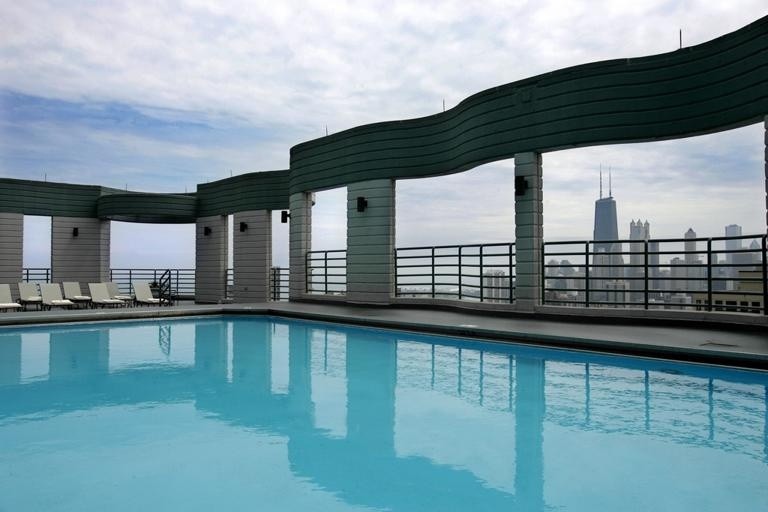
[88,283,126,309]
[18,282,42,311]
[62,282,92,309]
[131,280,169,307]
[39,283,74,311]
[105,282,135,308]
[0,284,22,313]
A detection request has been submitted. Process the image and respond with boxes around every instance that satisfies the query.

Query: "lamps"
[515,175,529,197]
[357,196,368,213]
[240,222,247,232]
[73,227,79,236]
[204,227,211,236]
[282,211,291,223]
